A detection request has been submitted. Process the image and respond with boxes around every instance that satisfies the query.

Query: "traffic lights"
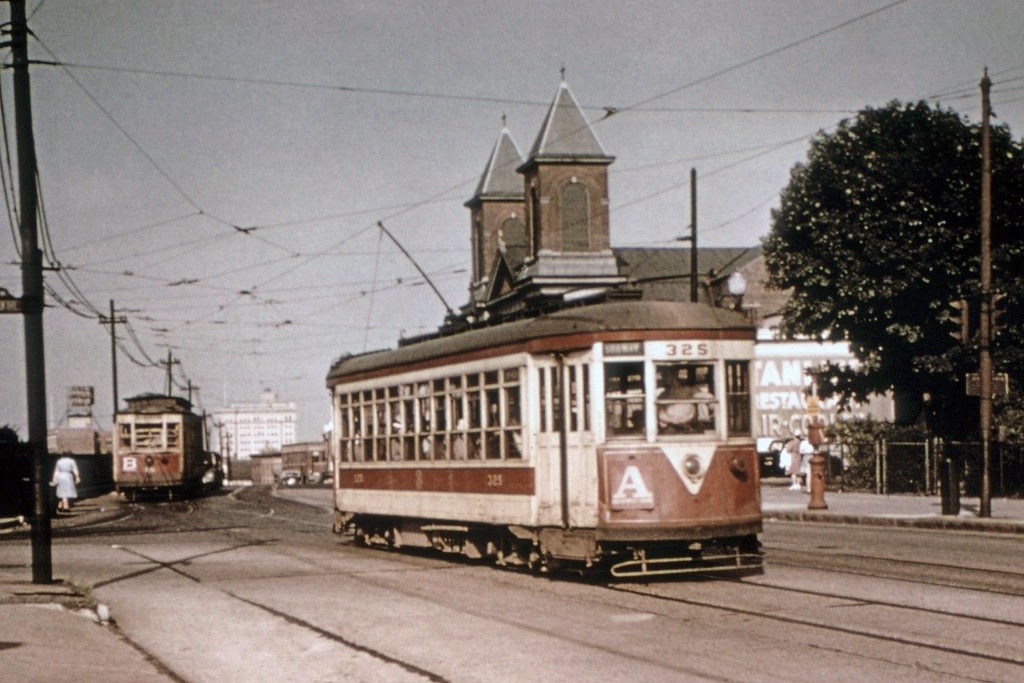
[990,290,1010,342]
[948,300,969,345]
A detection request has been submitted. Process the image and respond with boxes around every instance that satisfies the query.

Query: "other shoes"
[58,507,63,512]
[789,484,801,490]
[64,509,70,512]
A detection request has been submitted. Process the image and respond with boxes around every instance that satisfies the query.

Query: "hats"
[794,429,801,436]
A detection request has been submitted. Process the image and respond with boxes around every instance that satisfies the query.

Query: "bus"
[110,391,225,500]
[322,224,767,579]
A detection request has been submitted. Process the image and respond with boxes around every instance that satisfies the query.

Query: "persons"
[453,433,474,459]
[391,429,408,460]
[422,435,445,459]
[657,367,713,430]
[52,450,81,511]
[787,430,813,493]
